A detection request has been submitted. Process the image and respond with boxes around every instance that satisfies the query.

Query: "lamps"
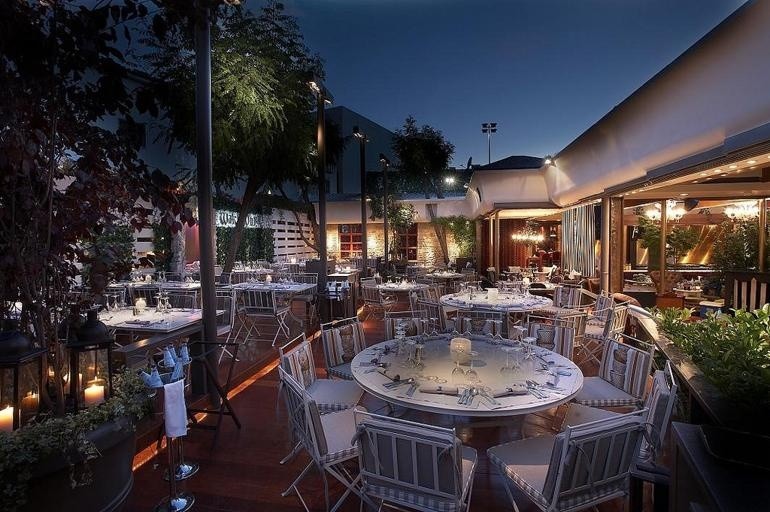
[511,220,544,244]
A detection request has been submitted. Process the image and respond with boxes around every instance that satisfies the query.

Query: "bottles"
[623,262,632,270]
[136,342,189,389]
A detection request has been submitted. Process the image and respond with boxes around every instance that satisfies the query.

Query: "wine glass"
[674,275,704,291]
[449,278,544,307]
[98,290,174,327]
[631,274,653,285]
[502,263,541,282]
[232,253,459,292]
[363,315,576,410]
[107,270,195,288]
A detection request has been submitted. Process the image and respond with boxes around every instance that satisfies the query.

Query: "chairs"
[0,257,280,395]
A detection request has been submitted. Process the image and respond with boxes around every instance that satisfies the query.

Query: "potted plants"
[0,367,156,512]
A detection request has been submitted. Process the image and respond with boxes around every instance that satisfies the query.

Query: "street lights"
[353,125,372,280]
[378,149,391,269]
[481,119,500,164]
[299,69,336,333]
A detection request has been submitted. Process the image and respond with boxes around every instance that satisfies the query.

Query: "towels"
[163,379,188,438]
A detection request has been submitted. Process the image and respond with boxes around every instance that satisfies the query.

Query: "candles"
[0,368,104,434]
